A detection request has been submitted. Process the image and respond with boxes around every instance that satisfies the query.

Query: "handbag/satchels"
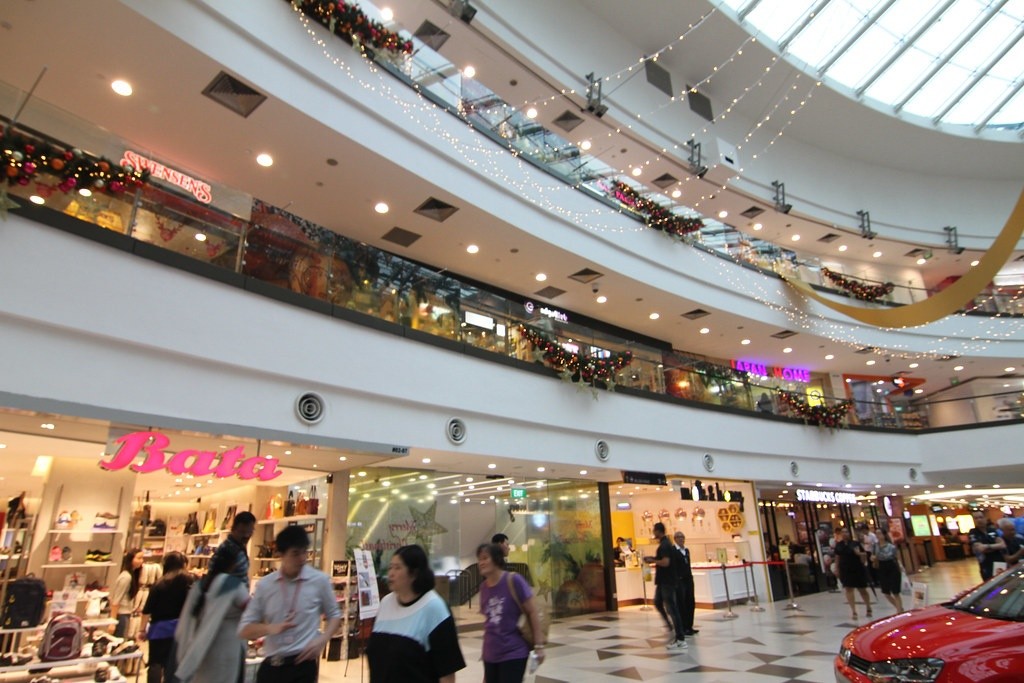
[182,486,319,534]
[146,616,178,639]
[643,562,651,582]
[900,570,912,597]
[506,570,550,648]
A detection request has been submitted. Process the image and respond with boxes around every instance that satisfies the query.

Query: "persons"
[939,523,951,538]
[208,511,256,683]
[365,545,467,683]
[491,534,510,556]
[613,537,635,567]
[175,546,253,683]
[757,392,773,413]
[142,551,199,683]
[642,522,699,648]
[238,526,341,683]
[783,523,904,619]
[967,512,1024,583]
[109,549,143,636]
[477,543,544,683]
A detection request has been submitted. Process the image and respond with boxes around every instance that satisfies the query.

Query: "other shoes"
[49,580,140,683]
[0,497,119,564]
[852,611,857,620]
[684,628,698,635]
[183,538,280,576]
[865,610,871,618]
[0,631,44,666]
[247,638,264,658]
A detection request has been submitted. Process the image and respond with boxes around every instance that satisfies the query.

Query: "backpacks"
[2,573,47,629]
[38,610,82,661]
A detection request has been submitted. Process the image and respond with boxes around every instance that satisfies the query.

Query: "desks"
[0,618,144,683]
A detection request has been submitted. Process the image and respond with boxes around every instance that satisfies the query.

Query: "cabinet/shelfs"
[127,490,326,598]
[323,560,361,662]
[0,485,126,611]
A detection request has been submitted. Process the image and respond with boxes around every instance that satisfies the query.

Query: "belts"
[266,654,296,667]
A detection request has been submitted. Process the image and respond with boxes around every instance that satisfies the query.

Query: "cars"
[834,560,1024,683]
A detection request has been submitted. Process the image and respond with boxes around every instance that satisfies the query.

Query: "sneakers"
[666,639,688,650]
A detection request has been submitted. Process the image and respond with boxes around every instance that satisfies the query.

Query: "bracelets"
[534,645,544,649]
